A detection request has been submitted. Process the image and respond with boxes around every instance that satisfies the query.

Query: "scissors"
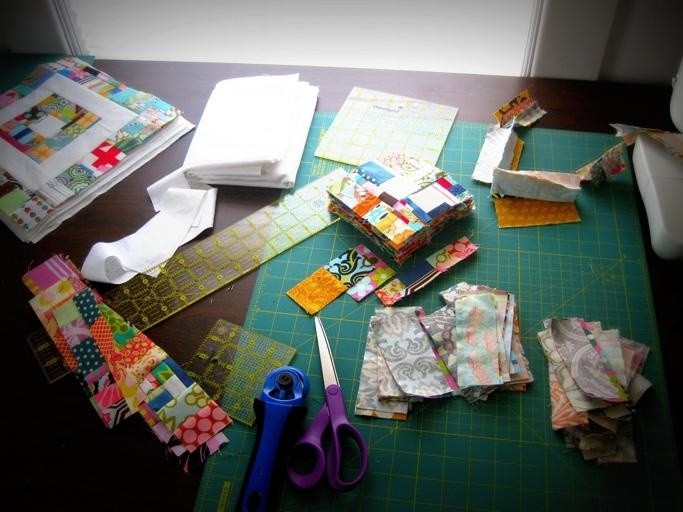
[285,315,368,489]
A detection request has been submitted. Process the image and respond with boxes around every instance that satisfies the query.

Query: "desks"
[0,55,683,512]
